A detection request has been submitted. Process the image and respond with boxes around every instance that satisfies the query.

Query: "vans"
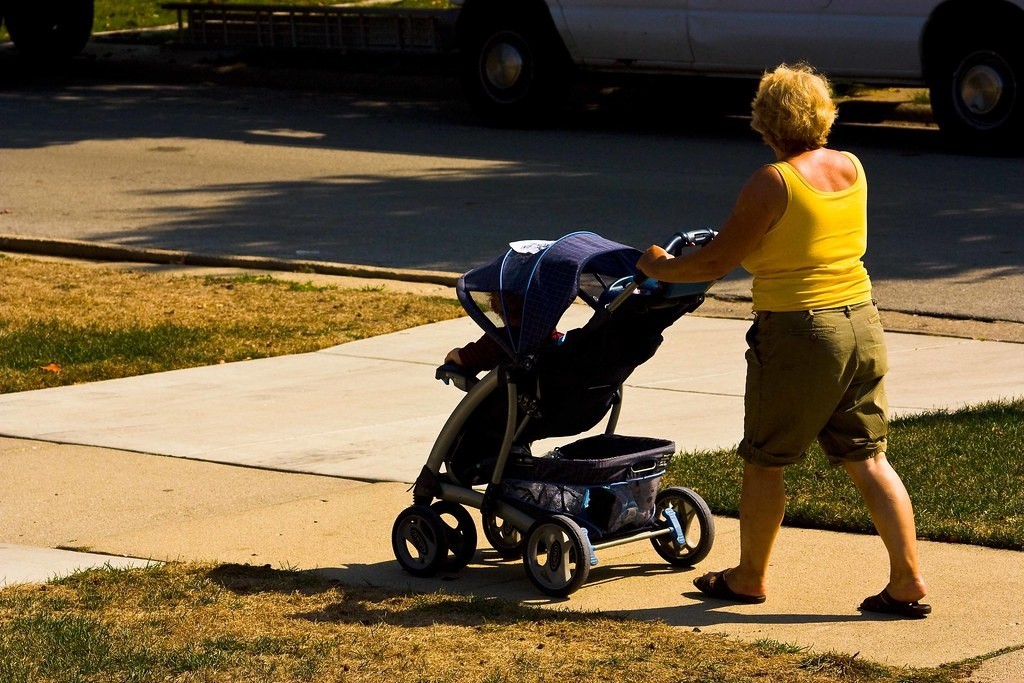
[452,0,1024,147]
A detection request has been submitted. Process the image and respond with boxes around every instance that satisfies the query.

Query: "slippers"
[693,572,766,603]
[860,589,932,615]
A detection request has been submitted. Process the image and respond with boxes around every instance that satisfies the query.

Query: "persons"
[636,59,932,618]
[444,284,564,370]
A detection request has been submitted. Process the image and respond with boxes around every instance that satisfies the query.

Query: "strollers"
[392,223,717,598]
